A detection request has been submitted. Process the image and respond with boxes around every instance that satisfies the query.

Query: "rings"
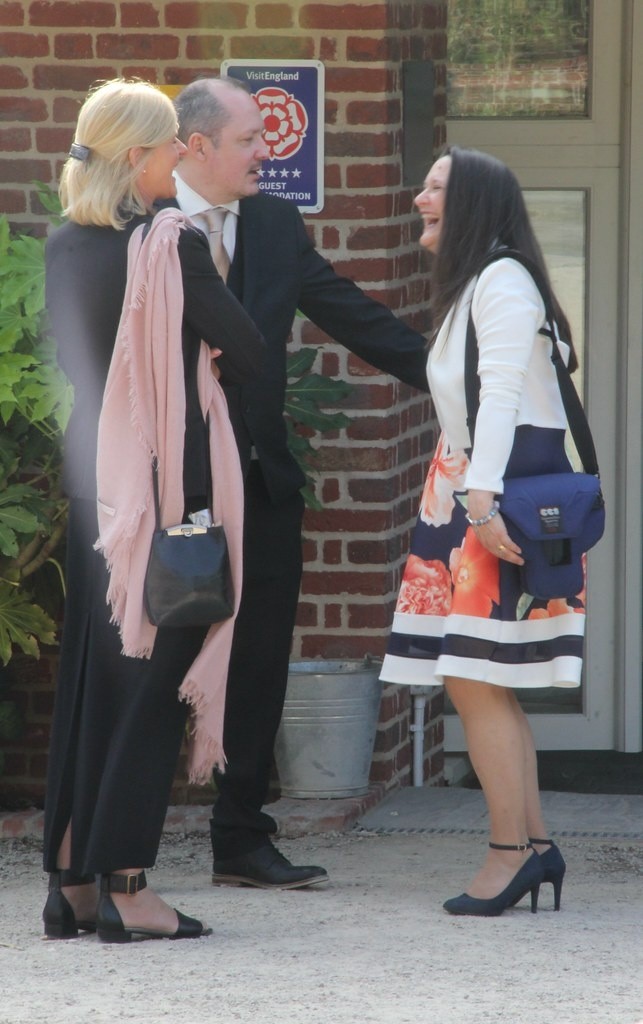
[498,545,506,551]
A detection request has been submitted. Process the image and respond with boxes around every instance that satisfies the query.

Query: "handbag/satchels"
[494,471,606,599]
[143,527,234,627]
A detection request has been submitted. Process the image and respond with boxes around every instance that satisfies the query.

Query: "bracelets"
[465,500,500,526]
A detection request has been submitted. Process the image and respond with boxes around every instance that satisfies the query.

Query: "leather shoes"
[211,845,327,889]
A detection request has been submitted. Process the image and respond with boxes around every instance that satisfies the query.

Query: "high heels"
[44,867,102,939]
[95,873,211,943]
[535,844,568,911]
[442,847,543,914]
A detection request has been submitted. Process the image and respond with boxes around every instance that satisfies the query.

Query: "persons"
[378,148,585,916]
[43,79,252,943]
[153,80,433,894]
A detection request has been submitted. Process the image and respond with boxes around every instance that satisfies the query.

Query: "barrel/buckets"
[273,657,384,798]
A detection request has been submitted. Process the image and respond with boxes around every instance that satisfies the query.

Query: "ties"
[199,208,232,282]
